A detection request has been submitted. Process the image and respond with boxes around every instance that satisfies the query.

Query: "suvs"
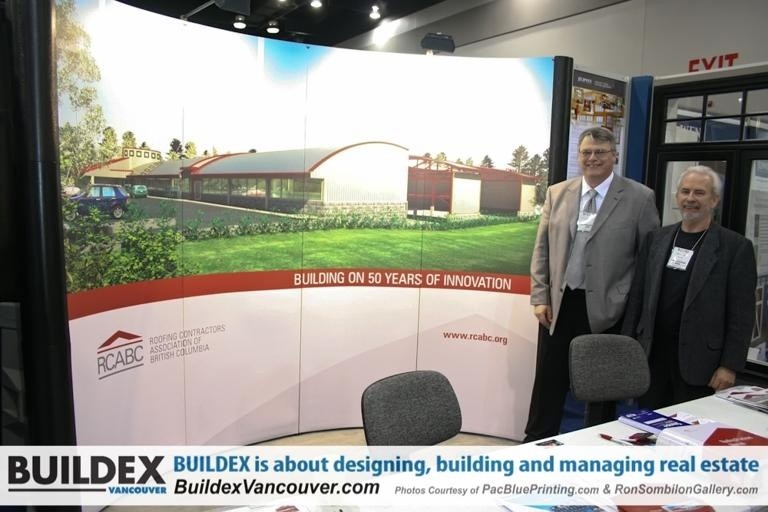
[133,185,148,199]
[72,183,131,219]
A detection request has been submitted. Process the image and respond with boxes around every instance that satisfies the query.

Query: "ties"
[564,190,598,290]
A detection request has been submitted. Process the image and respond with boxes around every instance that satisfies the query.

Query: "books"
[713,384,768,414]
[617,408,714,436]
[608,504,716,512]
[503,504,620,512]
[655,421,768,446]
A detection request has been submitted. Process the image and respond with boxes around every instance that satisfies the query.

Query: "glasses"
[579,148,613,158]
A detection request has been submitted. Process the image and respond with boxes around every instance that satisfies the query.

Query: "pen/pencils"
[601,434,633,446]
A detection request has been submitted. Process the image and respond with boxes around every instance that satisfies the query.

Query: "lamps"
[419,33,457,55]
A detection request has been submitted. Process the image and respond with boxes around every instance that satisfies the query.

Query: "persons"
[518,129,663,448]
[623,166,756,412]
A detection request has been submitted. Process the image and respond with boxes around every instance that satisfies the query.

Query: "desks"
[223,384,767,511]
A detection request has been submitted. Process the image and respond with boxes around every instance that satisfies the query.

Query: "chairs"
[363,370,463,446]
[568,331,654,429]
